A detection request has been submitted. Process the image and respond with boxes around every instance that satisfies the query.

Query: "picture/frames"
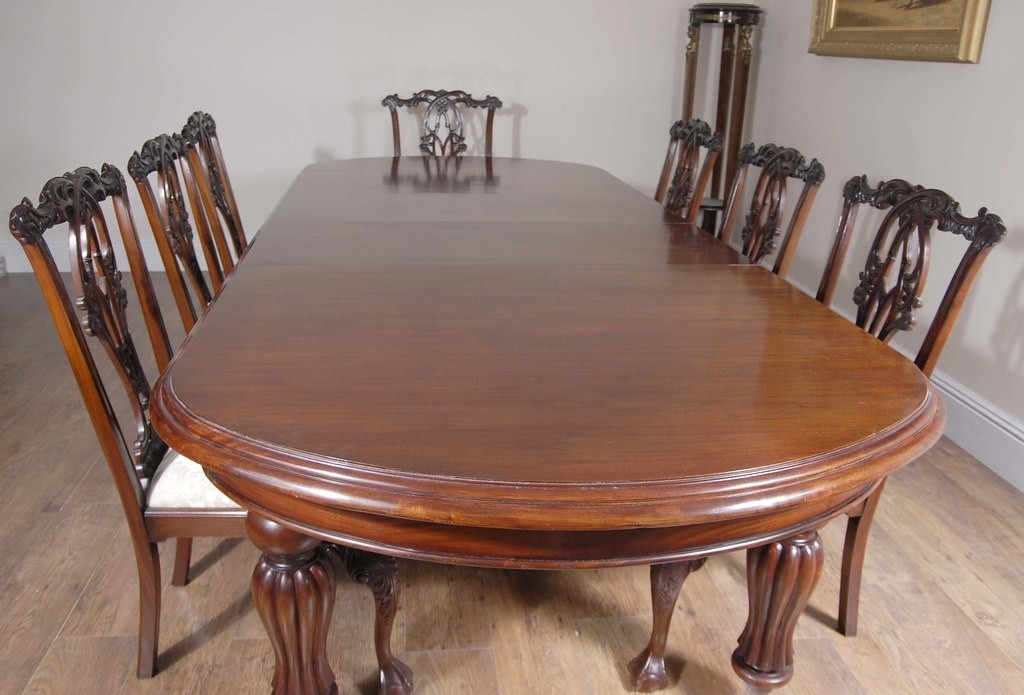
[807,0,991,65]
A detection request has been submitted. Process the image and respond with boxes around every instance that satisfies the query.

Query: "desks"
[149,154,949,695]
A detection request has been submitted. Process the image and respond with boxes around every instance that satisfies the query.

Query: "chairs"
[127,111,249,338]
[382,88,503,158]
[684,173,1007,636]
[7,162,413,695]
[715,141,825,280]
[652,117,723,228]
